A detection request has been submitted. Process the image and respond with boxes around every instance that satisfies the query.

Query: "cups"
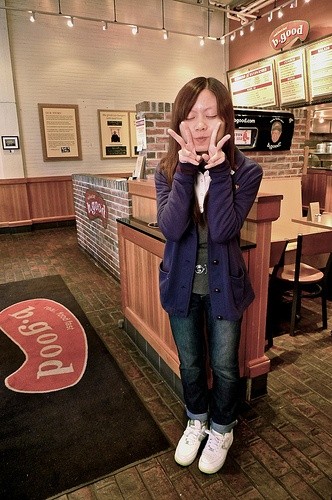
[317,144,326,153]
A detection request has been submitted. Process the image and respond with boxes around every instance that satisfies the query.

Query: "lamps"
[199,38,205,48]
[267,12,273,23]
[163,30,168,40]
[240,27,245,36]
[103,22,108,30]
[250,21,256,32]
[231,31,237,41]
[132,26,139,35]
[289,0,297,9]
[67,17,73,27]
[277,7,285,19]
[29,11,37,23]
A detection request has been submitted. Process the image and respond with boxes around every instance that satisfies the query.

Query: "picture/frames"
[1,136,20,150]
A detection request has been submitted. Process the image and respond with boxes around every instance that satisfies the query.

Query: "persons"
[154,76,263,474]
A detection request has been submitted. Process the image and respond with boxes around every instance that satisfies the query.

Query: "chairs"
[267,237,289,347]
[269,230,332,338]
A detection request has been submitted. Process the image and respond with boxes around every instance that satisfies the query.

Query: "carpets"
[0,274,175,500]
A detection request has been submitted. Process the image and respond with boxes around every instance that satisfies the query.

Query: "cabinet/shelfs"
[301,108,332,213]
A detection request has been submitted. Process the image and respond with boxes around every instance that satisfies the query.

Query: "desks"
[291,212,332,302]
[115,213,257,419]
[270,219,332,323]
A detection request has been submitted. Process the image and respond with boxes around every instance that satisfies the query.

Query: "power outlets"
[9,150,15,153]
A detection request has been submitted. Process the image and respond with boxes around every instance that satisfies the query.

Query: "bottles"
[326,143,332,153]
[315,214,322,222]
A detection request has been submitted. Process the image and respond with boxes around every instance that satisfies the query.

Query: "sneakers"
[198,426,234,474]
[174,420,208,466]
